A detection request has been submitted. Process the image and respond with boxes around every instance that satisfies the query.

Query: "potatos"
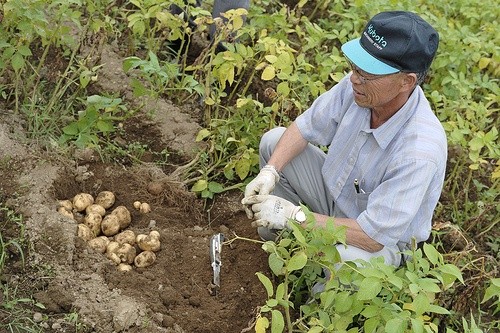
[57,190,160,272]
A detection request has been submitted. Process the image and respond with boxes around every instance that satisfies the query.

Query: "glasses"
[343,54,389,81]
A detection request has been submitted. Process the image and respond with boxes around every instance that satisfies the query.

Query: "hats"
[341,10,440,77]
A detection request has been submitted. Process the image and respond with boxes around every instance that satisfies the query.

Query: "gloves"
[242,165,281,220]
[241,194,302,232]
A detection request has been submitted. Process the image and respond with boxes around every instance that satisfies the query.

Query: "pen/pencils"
[354,178,360,197]
